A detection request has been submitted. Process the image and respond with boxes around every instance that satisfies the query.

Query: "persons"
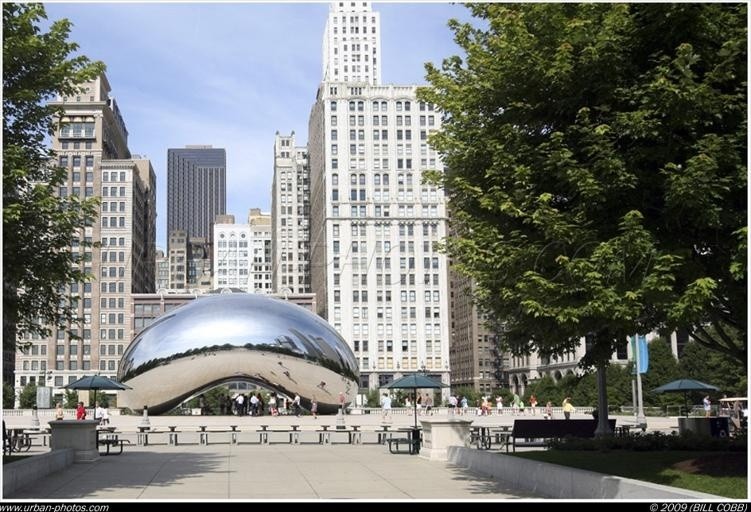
[76,400,87,419]
[379,392,434,425]
[719,392,731,416]
[56,402,65,419]
[544,411,553,419]
[704,394,712,416]
[102,403,112,423]
[447,393,539,415]
[199,392,206,415]
[547,398,553,419]
[562,393,578,419]
[218,390,319,420]
[339,391,347,416]
[96,400,104,422]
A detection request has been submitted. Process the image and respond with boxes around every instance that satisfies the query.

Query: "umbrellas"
[651,378,723,417]
[377,372,453,427]
[59,375,136,419]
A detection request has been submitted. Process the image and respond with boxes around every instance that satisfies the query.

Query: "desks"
[383,428,423,454]
[94,427,125,456]
[471,424,512,450]
[5,425,42,451]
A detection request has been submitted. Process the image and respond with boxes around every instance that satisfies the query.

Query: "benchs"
[469,431,511,450]
[5,436,130,456]
[387,437,421,454]
[504,418,616,455]
